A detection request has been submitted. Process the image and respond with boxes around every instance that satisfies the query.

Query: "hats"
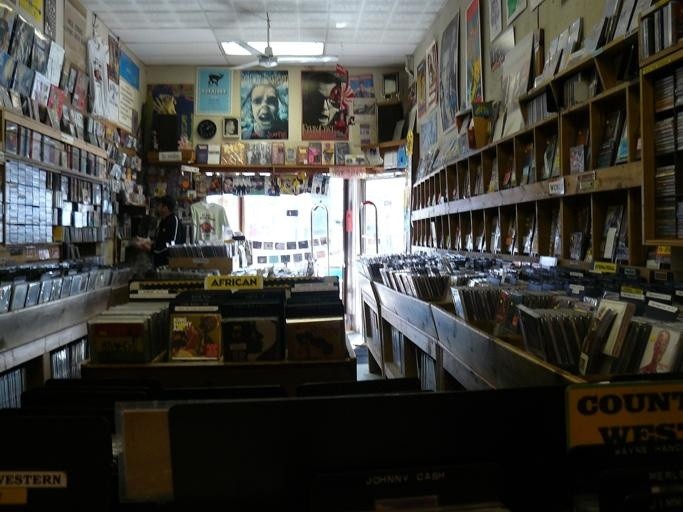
[154,195,176,209]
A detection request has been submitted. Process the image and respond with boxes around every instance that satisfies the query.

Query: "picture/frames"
[193,67,233,116]
[222,117,240,138]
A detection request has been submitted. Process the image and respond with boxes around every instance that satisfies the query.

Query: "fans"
[223,11,339,72]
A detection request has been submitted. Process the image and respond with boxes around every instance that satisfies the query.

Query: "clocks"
[197,120,216,139]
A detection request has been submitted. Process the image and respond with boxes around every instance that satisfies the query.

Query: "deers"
[208,72,224,87]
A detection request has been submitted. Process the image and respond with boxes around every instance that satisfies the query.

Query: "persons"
[136,195,184,276]
[435,42,458,131]
[302,80,340,131]
[224,121,235,135]
[356,80,373,98]
[239,81,286,140]
[641,330,672,373]
